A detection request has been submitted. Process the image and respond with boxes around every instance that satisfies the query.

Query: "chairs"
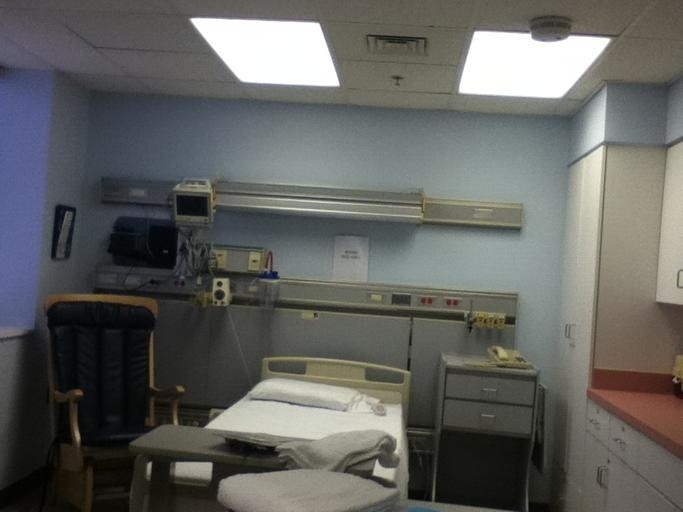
[38,291,188,512]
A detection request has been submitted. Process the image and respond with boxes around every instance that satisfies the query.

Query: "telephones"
[487,345,528,368]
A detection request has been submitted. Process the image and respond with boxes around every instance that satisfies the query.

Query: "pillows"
[245,372,362,412]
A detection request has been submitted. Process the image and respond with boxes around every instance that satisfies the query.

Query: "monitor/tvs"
[172,178,216,223]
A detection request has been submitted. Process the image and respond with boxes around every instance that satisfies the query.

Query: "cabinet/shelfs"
[651,141,682,307]
[544,145,682,511]
[575,395,640,512]
[427,350,541,511]
[640,433,683,512]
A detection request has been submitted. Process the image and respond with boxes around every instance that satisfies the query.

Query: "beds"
[123,350,416,510]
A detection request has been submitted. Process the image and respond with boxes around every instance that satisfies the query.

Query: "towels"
[213,459,400,510]
[271,420,403,479]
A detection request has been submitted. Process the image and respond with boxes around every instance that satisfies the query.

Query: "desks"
[127,420,376,510]
